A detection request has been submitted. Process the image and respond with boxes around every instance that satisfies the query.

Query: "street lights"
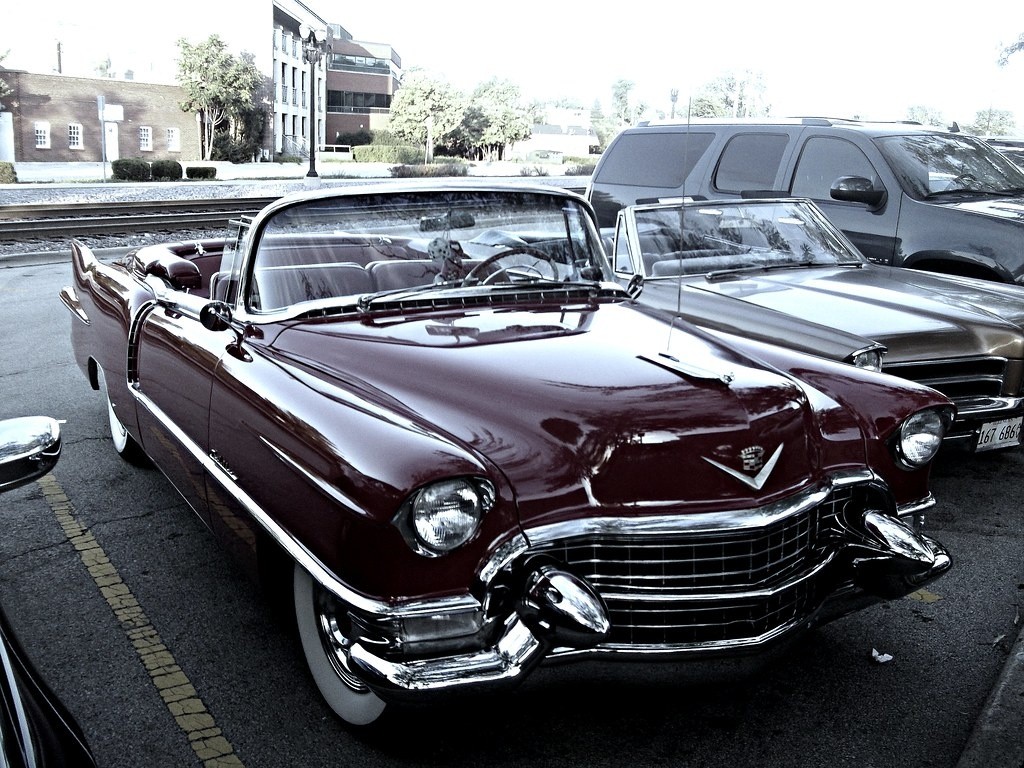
[296,20,328,177]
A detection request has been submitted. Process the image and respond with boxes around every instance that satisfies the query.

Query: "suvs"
[581,117,1024,289]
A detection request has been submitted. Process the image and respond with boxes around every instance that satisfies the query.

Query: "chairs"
[576,253,662,278]
[367,259,513,293]
[662,248,732,262]
[210,262,374,312]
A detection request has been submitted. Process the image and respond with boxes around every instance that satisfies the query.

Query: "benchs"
[181,244,397,300]
[527,233,680,262]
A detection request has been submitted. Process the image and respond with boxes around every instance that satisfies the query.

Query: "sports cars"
[55,183,959,747]
[459,194,1023,458]
